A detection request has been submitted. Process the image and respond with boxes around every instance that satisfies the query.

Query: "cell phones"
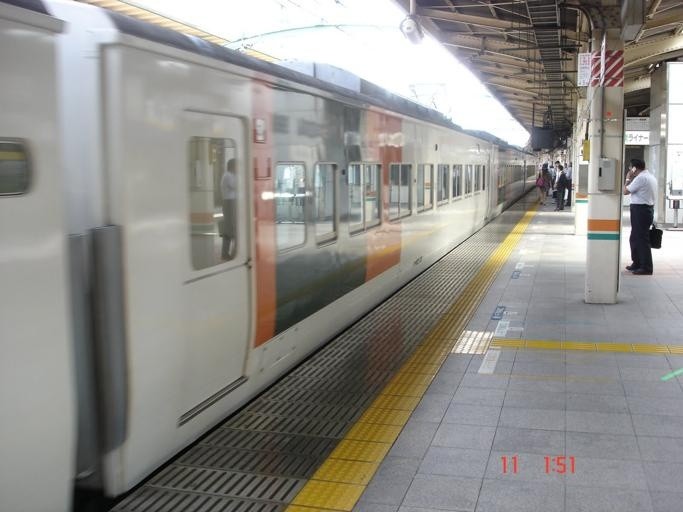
[632,167,637,173]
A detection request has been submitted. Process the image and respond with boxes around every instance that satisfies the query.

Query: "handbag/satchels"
[648,224,664,249]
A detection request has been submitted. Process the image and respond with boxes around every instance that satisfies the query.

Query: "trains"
[0,0,536,510]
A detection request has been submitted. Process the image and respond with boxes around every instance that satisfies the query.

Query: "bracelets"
[624,178,630,186]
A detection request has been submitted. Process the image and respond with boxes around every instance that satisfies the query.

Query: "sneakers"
[626,263,641,271]
[632,267,653,275]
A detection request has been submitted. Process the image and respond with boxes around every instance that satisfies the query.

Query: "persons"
[622,157,658,275]
[534,158,572,211]
[215,157,238,261]
